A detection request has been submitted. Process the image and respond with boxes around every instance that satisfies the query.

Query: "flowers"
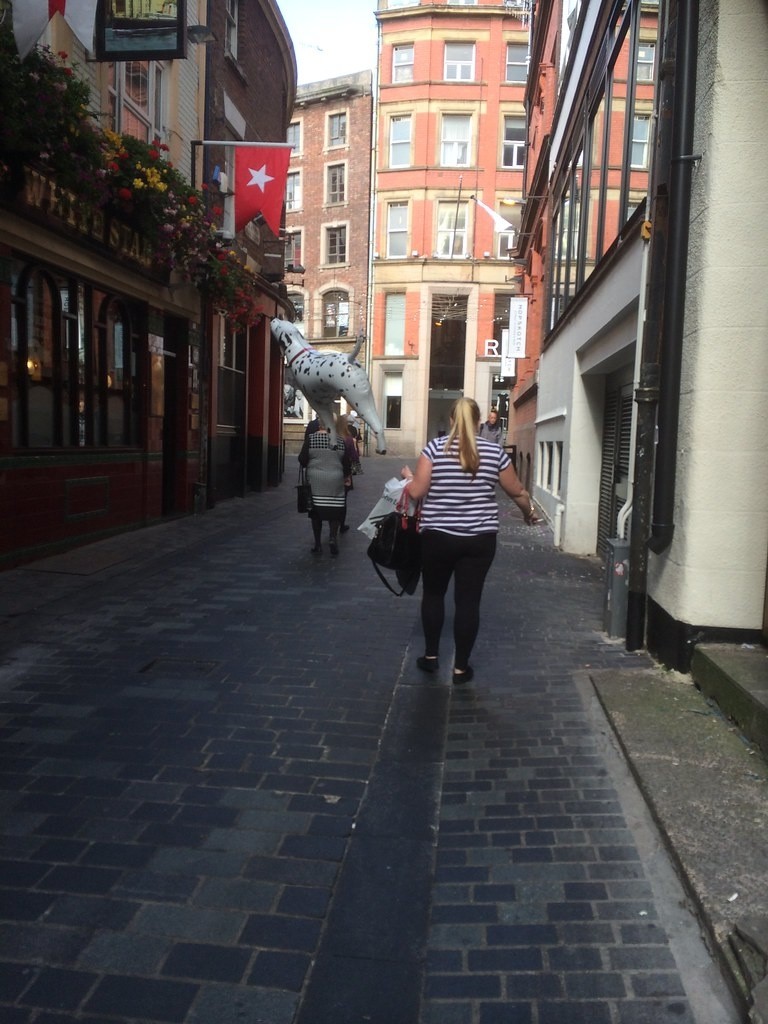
[0,35,266,336]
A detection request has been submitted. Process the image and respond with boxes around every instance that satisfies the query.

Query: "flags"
[231,145,292,238]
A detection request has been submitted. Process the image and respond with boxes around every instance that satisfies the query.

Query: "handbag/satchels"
[357,477,420,598]
[295,463,314,513]
[351,460,363,475]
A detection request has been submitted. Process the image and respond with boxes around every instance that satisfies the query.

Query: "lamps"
[432,250,439,259]
[483,251,490,259]
[373,252,380,260]
[411,249,419,258]
[284,263,305,274]
[464,252,471,259]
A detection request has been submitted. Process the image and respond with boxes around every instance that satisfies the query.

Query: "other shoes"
[340,526,349,533]
[416,656,440,669]
[311,545,322,554]
[452,667,474,684]
[329,537,339,555]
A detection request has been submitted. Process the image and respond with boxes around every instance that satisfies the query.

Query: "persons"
[437,417,446,437]
[402,397,542,683]
[478,411,503,448]
[299,410,361,555]
